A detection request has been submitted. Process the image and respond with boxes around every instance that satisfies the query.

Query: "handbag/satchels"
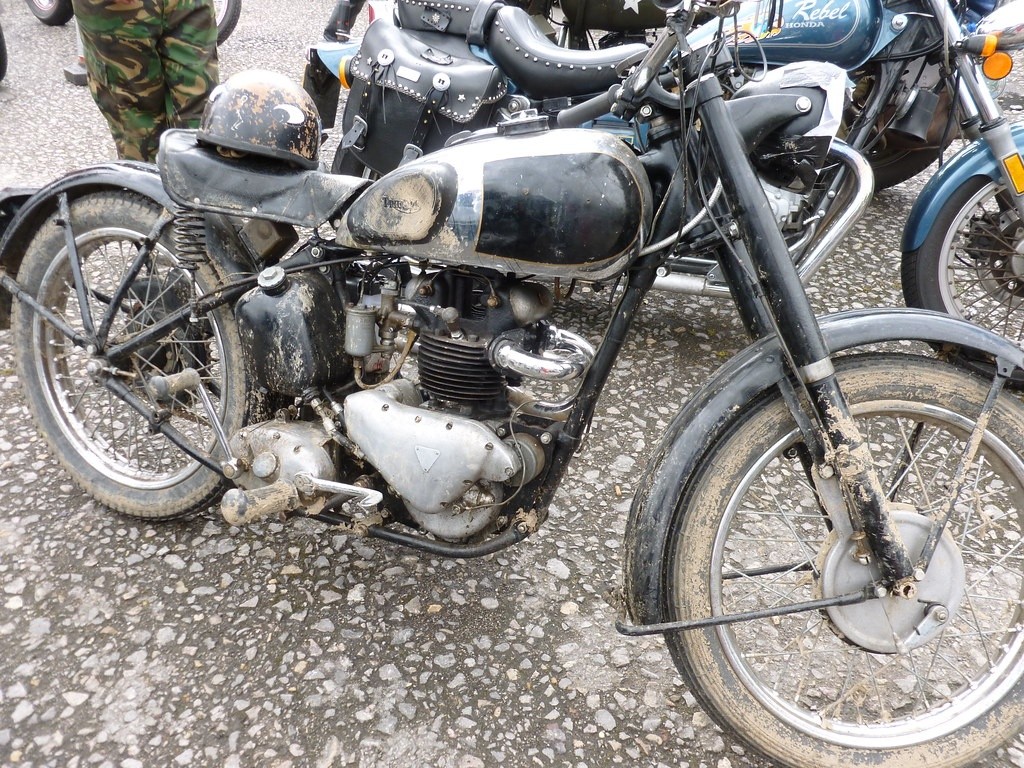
[341,17,506,176]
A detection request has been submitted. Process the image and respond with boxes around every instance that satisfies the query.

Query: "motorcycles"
[302,1,1022,395]
[1,1,1022,766]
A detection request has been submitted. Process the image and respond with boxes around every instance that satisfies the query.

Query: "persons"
[68,0,220,170]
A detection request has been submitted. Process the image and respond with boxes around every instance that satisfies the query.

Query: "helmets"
[197,69,320,171]
